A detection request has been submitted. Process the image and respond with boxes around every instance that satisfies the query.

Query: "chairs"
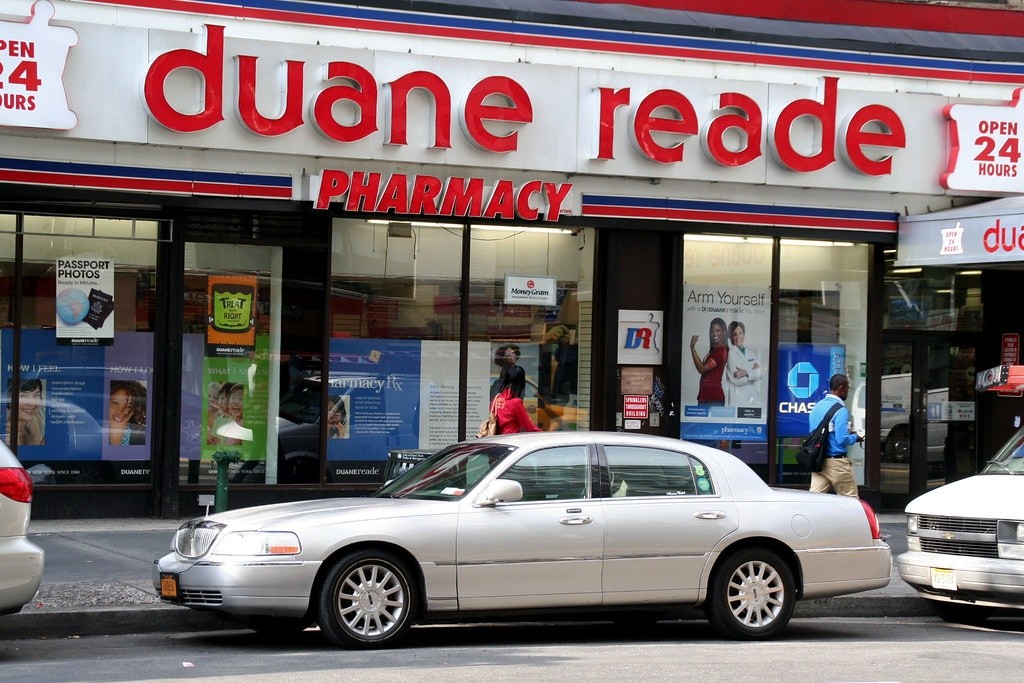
[510,459,536,486]
[640,455,671,496]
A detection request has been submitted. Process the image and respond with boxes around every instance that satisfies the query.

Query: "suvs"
[928,386,949,476]
[898,425,1024,623]
[881,373,911,462]
[219,371,421,487]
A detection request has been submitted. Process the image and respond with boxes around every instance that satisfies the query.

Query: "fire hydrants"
[211,450,242,512]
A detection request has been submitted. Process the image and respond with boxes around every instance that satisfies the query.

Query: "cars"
[491,377,590,431]
[0,396,146,462]
[152,388,892,650]
[0,438,46,616]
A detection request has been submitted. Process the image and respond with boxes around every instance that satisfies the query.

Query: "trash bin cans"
[381,450,459,492]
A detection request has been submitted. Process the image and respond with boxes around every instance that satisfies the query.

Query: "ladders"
[976,365,1024,391]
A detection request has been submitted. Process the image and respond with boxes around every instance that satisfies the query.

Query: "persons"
[690,318,729,406]
[489,365,543,466]
[810,374,861,499]
[109,380,146,445]
[6,378,45,445]
[206,381,244,446]
[722,320,762,406]
[327,395,348,438]
[494,343,521,379]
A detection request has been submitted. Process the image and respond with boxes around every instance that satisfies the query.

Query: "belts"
[822,456,843,458]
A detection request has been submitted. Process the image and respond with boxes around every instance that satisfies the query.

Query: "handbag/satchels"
[796,403,844,472]
[476,392,502,439]
